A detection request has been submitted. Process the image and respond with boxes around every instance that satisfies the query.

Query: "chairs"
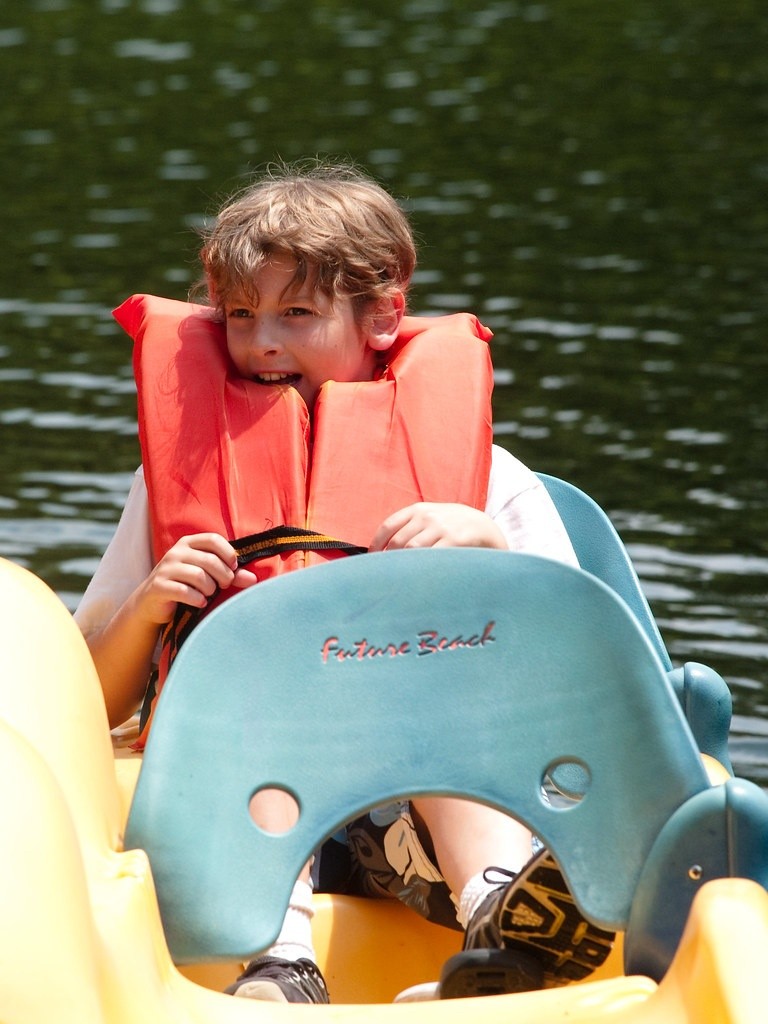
[522,465,673,677]
[114,547,712,1006]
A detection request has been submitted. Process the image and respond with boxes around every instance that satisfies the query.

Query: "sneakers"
[463,843,615,991]
[223,954,329,1006]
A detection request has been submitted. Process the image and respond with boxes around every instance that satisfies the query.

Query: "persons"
[74,154,616,1004]
